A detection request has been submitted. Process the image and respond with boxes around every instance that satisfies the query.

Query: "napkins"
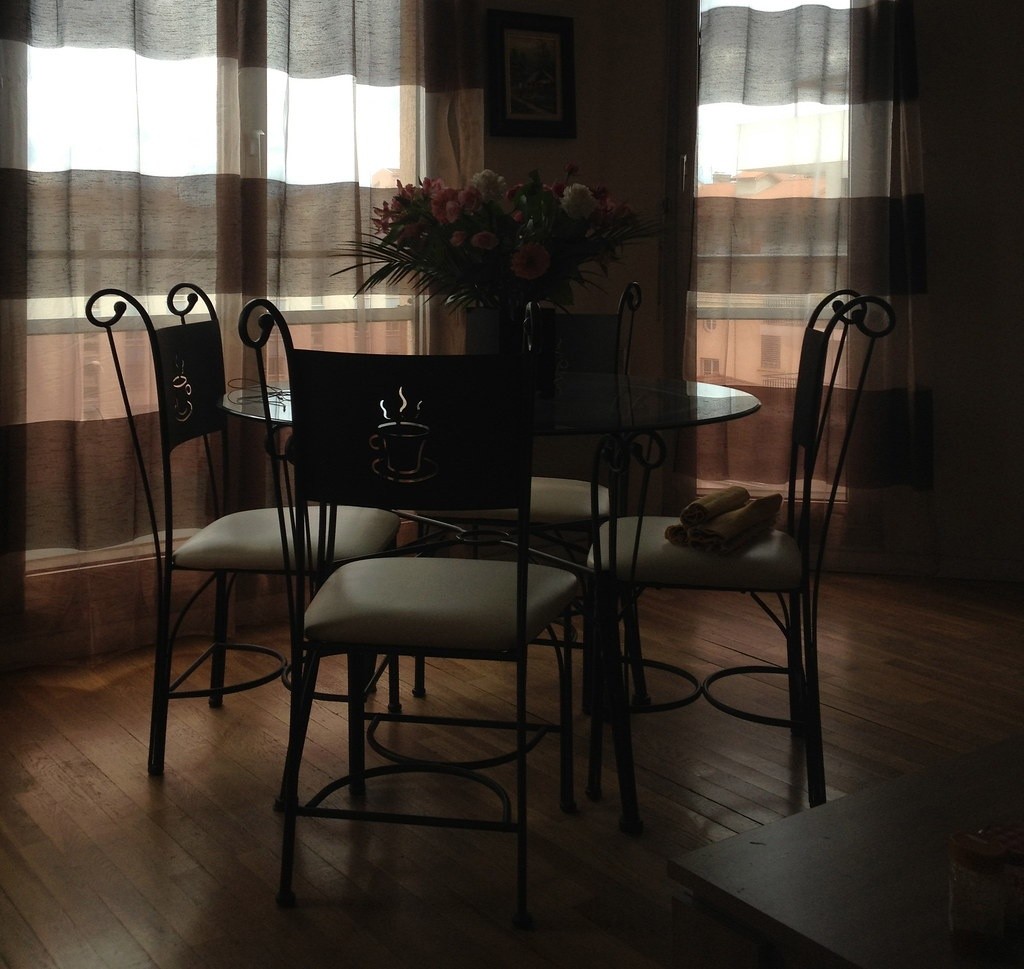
[664,486,783,554]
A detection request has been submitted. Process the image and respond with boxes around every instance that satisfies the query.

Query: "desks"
[214,371,762,834]
[663,732,1023,969]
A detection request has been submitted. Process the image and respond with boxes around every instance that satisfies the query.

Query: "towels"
[664,487,783,552]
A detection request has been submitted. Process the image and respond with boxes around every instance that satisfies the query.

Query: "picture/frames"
[484,8,577,139]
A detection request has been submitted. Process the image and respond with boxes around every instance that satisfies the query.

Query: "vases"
[464,307,555,399]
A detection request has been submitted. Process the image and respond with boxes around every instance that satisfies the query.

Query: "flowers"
[329,164,671,313]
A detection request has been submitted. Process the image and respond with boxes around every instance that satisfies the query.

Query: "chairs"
[85,283,895,928]
